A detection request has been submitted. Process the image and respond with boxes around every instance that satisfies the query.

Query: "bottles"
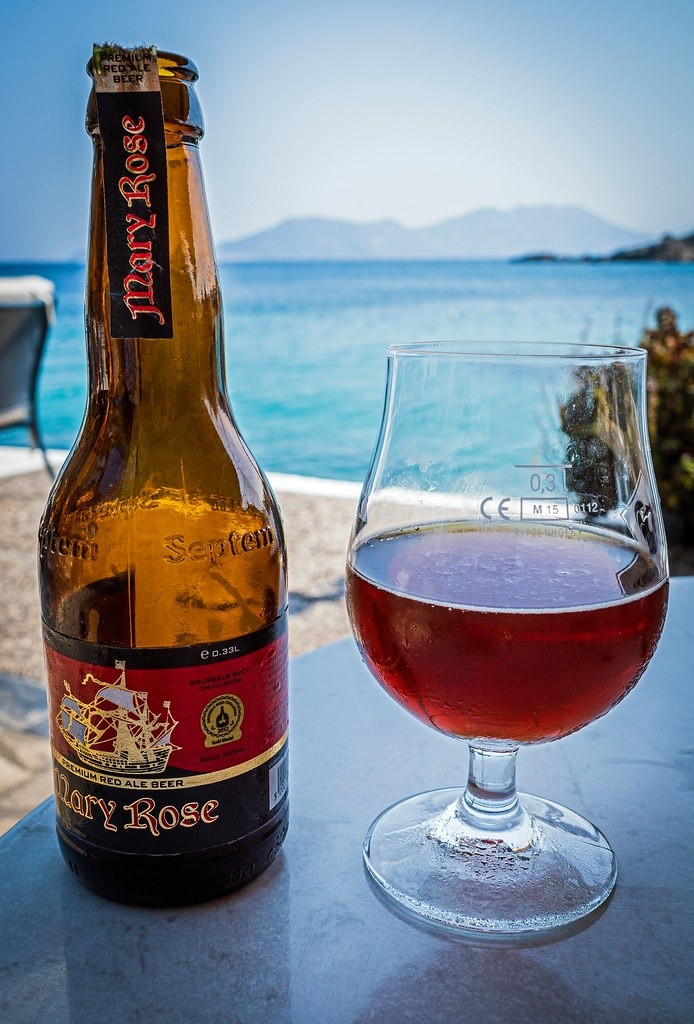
[37,49,290,908]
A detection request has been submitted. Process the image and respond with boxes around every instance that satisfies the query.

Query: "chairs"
[0,274,61,479]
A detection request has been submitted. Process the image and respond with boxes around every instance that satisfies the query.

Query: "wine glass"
[342,337,672,942]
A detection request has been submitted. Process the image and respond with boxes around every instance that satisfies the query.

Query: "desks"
[0,576,694,1024]
